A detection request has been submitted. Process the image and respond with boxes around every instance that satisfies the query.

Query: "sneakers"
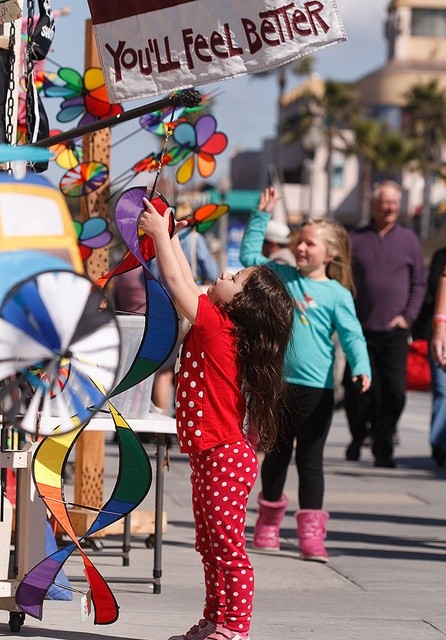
[205,626,248,640]
[169,618,217,640]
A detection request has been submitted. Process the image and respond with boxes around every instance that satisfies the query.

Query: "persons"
[336,179,429,469]
[434,252,446,369]
[137,193,295,640]
[237,181,372,564]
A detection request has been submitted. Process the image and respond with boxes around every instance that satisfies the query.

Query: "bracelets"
[434,308,446,329]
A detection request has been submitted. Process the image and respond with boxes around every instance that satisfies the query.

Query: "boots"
[255,491,287,552]
[296,510,329,562]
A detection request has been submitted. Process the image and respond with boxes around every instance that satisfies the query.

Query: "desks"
[30,409,179,596]
